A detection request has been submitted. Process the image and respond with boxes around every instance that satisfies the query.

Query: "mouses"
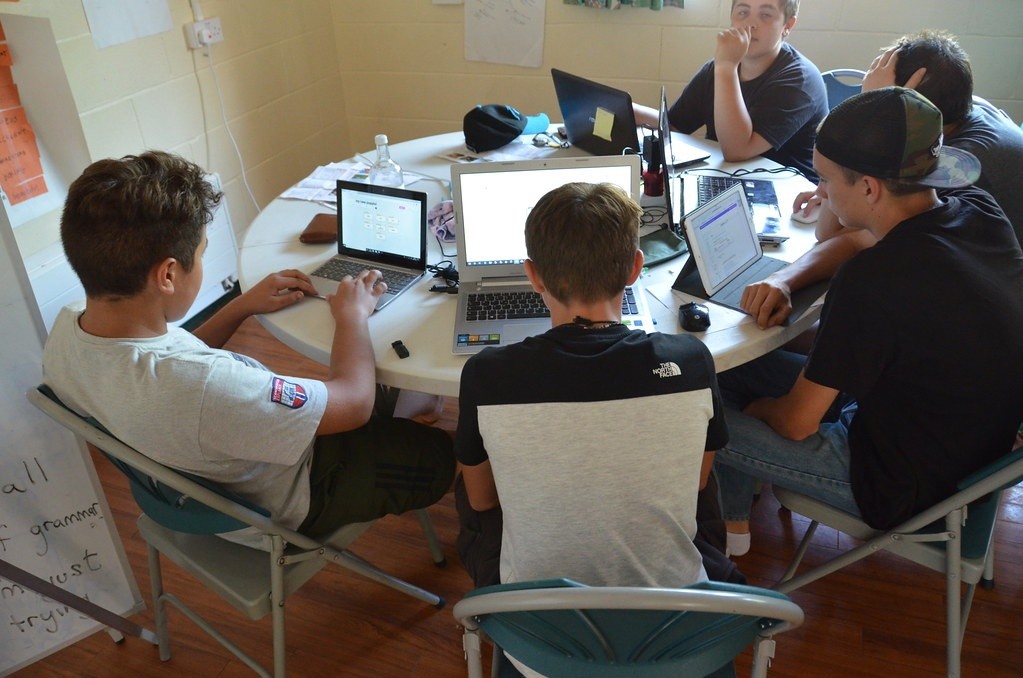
[680,301,711,332]
[791,204,821,224]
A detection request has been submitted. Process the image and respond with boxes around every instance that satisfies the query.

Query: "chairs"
[821,69,867,112]
[771,444,1023,678]
[25,383,448,678]
[452,578,804,678]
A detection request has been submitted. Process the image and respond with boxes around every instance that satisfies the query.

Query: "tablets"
[679,182,763,298]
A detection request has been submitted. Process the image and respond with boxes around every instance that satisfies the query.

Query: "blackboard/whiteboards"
[0,206,151,678]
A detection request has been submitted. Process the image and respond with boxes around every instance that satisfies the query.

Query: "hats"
[463,104,550,154]
[814,85,982,188]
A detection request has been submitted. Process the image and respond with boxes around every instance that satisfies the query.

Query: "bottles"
[368,133,405,190]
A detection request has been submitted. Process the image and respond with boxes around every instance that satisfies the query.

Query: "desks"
[238,123,827,400]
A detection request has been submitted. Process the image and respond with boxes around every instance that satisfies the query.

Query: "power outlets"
[185,16,224,49]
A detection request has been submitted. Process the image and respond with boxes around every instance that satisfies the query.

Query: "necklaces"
[561,316,618,329]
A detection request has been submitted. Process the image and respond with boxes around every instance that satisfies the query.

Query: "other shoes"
[725,530,750,559]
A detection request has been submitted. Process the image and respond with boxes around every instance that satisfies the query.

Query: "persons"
[632,0,830,186]
[712,85,1023,566]
[795,32,1023,243]
[43,150,460,552]
[459,183,726,590]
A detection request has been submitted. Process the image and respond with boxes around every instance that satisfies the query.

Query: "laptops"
[289,179,428,312]
[449,68,790,356]
